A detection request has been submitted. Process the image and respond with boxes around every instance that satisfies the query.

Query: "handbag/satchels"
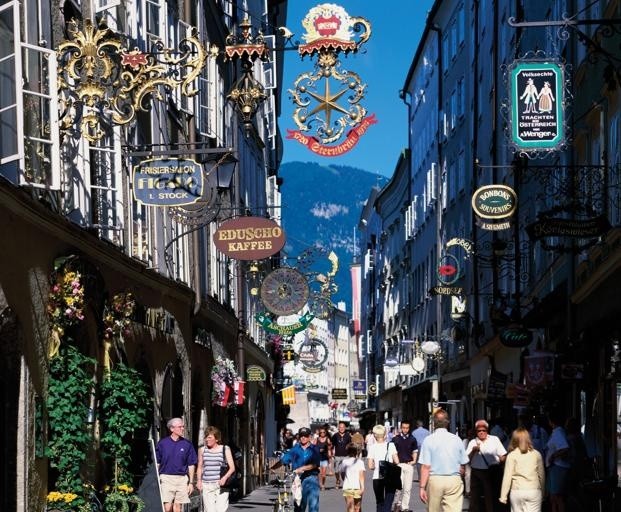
[487,463,503,484]
[220,461,242,489]
[565,452,585,484]
[380,461,402,494]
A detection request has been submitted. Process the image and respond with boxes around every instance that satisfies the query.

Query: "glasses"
[477,429,486,433]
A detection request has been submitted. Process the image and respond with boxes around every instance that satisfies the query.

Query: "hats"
[299,427,311,437]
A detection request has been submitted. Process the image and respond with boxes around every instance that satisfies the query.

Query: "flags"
[282,385,297,405]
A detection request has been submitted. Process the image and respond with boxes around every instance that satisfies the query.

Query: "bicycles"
[263,467,306,512]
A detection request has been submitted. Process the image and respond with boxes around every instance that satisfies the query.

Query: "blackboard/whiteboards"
[100,438,165,512]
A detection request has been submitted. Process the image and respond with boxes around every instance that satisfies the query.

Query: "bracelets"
[190,481,194,484]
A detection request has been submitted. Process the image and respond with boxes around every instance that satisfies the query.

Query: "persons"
[536,81,556,114]
[276,410,586,511]
[519,76,540,113]
[156,416,196,512]
[195,426,237,512]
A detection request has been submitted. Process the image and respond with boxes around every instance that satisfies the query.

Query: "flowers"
[208,355,248,408]
[41,269,140,339]
